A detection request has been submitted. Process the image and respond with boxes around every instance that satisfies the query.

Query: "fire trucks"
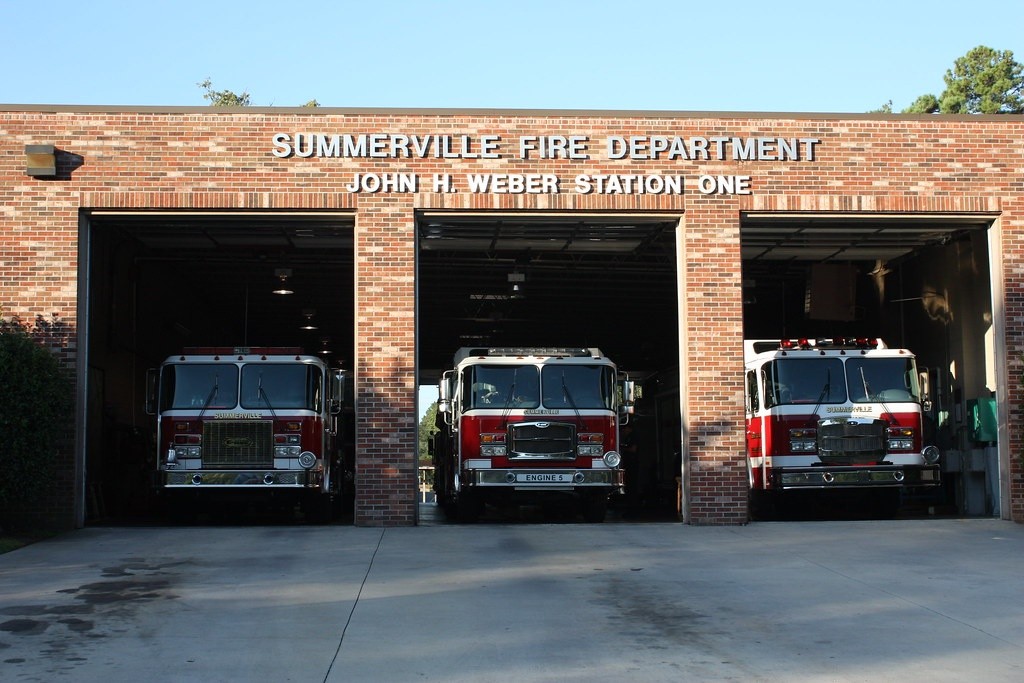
[743,331,942,517]
[431,345,641,519]
[142,344,347,524]
